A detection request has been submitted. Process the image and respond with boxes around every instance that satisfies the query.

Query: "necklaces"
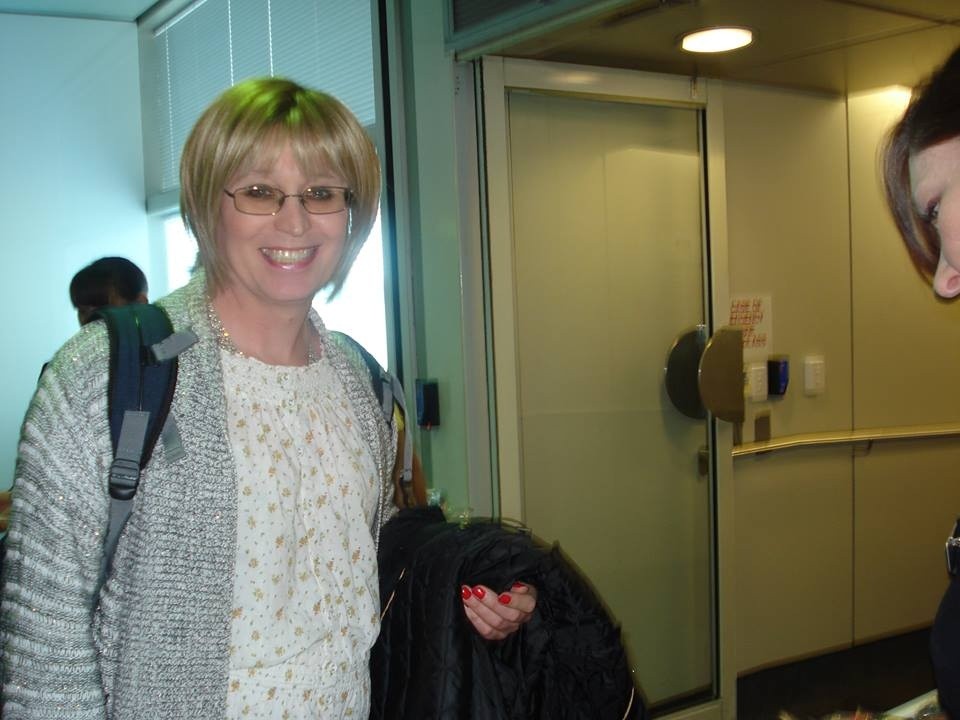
[206,299,317,367]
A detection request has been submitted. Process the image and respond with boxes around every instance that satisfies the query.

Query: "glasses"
[223,183,356,216]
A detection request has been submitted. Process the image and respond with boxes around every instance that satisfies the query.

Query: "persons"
[884,47,960,720]
[70,257,148,325]
[0,80,537,719]
